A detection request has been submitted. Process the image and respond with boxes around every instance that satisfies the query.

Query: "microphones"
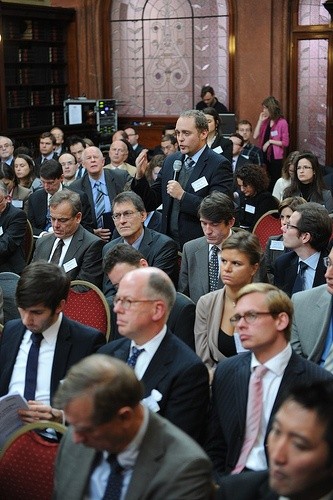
[170,160,183,198]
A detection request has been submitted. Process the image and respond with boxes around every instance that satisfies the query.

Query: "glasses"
[229,309,280,326]
[323,257,333,267]
[297,166,313,170]
[112,211,142,220]
[286,220,307,233]
[112,296,163,310]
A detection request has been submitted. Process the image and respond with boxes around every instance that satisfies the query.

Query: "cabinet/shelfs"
[0,1,77,130]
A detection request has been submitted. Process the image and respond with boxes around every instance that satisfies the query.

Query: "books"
[3,18,68,128]
[0,391,30,450]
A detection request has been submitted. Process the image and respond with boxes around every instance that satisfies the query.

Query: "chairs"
[62,280,111,341]
[0,421,70,500]
[252,210,283,253]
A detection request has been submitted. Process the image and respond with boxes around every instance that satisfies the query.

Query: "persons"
[290,236,333,375]
[196,86,228,113]
[209,283,333,475]
[0,261,107,425]
[194,231,260,384]
[275,202,331,298]
[96,267,209,451]
[204,96,333,233]
[130,110,233,246]
[0,125,180,242]
[54,354,218,500]
[101,191,179,304]
[0,182,28,275]
[31,191,104,292]
[105,243,196,352]
[178,193,235,303]
[219,376,333,500]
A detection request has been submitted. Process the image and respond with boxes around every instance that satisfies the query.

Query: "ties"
[101,454,125,500]
[50,239,65,266]
[186,157,195,167]
[24,332,44,401]
[229,366,269,474]
[126,346,145,371]
[209,245,220,292]
[290,261,309,296]
[95,181,106,228]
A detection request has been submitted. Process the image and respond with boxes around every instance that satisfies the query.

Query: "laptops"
[218,113,236,137]
